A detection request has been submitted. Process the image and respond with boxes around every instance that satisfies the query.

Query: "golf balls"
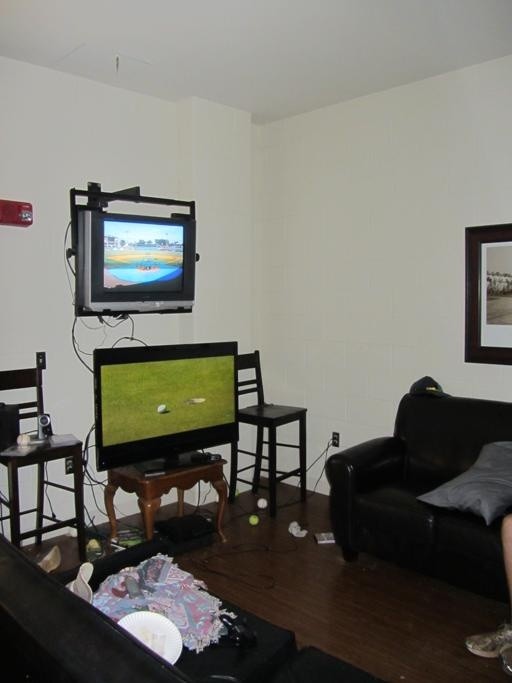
[158,404,166,413]
[17,434,30,446]
[258,498,268,509]
[249,515,259,525]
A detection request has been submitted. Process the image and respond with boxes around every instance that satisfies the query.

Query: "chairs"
[0,364,86,564]
[227,349,308,522]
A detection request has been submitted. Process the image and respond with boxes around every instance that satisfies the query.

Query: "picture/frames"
[464,222,512,366]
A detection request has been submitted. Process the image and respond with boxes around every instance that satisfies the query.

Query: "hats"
[65,562,95,605]
[411,375,448,397]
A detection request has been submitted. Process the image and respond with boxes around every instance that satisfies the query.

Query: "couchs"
[325,386,511,601]
[1,532,412,682]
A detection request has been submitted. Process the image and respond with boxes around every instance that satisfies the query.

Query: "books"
[48,434,80,447]
[1,445,38,457]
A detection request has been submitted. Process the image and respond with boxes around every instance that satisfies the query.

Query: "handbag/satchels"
[221,616,257,646]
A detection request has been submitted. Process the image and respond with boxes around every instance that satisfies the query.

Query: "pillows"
[419,440,512,523]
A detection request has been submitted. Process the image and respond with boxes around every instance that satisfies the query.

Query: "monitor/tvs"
[92,341,239,472]
[77,210,196,315]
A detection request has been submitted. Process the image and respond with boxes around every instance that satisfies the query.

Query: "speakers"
[37,413,51,440]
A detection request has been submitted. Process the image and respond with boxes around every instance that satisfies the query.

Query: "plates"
[116,610,184,668]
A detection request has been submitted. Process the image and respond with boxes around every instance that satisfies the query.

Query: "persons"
[485,271,512,298]
[465,511,511,677]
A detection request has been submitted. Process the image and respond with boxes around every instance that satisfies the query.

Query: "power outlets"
[63,457,75,474]
[330,431,341,448]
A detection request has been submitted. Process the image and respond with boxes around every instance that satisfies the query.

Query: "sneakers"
[499,643,511,675]
[36,545,62,573]
[465,622,511,658]
[85,538,106,562]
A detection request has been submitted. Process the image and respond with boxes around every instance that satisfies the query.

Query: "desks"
[101,451,229,548]
